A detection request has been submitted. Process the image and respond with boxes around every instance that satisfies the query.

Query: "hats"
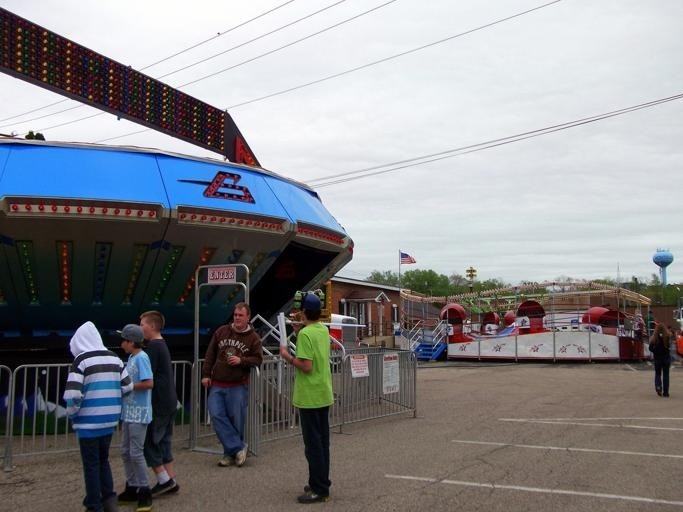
[297,292,322,311]
[114,323,145,344]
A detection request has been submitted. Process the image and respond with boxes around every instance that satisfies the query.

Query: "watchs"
[291,357,295,365]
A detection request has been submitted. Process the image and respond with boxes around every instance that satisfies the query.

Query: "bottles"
[227,353,233,357]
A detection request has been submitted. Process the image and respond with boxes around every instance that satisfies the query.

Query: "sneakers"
[297,492,330,503]
[235,443,249,467]
[167,479,180,493]
[304,485,312,490]
[135,499,151,511]
[152,476,175,499]
[217,455,233,467]
[119,488,137,500]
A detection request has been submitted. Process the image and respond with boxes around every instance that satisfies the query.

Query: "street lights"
[468,283,474,292]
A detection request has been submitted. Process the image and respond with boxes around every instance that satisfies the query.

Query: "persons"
[63,320,135,512]
[201,303,264,467]
[278,294,335,504]
[117,325,154,511]
[648,323,672,397]
[139,311,180,498]
